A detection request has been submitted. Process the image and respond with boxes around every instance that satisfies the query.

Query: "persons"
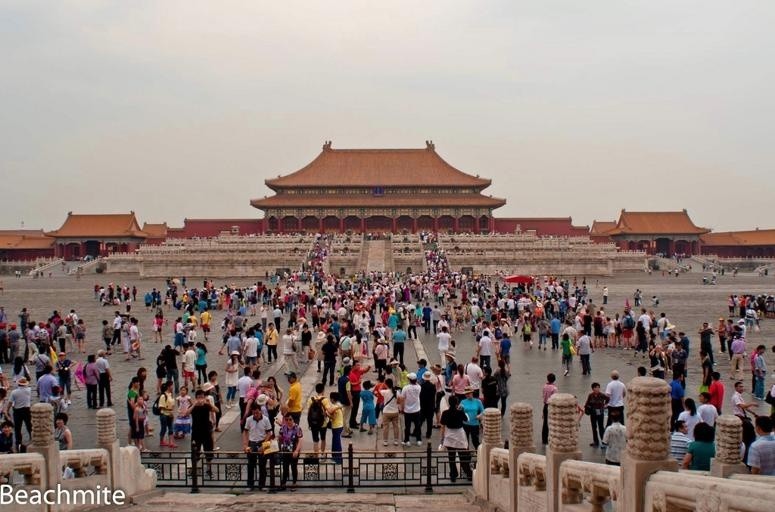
[2,219,770,494]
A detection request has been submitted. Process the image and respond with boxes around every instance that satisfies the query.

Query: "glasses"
[285,418,292,422]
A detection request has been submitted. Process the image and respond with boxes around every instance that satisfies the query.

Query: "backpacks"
[494,326,503,340]
[625,315,633,329]
[153,396,161,416]
[308,399,325,430]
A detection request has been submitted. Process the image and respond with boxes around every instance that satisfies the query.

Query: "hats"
[59,352,66,356]
[462,386,475,394]
[230,351,241,357]
[430,363,442,373]
[11,322,17,330]
[0,322,7,329]
[39,322,45,328]
[201,382,214,392]
[407,373,417,380]
[377,337,386,344]
[719,317,725,321]
[284,371,296,379]
[421,371,433,381]
[417,359,427,365]
[342,357,352,366]
[317,331,326,339]
[52,386,64,393]
[445,351,456,360]
[737,319,744,323]
[256,394,270,406]
[16,376,30,387]
[610,368,620,378]
[390,358,400,365]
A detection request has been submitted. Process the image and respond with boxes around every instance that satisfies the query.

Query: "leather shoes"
[349,431,353,435]
[342,435,352,438]
[356,423,361,426]
[350,426,359,429]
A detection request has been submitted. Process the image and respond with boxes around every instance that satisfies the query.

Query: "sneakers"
[417,441,424,447]
[276,488,287,491]
[319,454,326,461]
[406,441,411,447]
[246,487,254,492]
[393,441,398,446]
[564,369,569,376]
[566,372,570,376]
[383,441,389,446]
[67,400,72,405]
[260,488,267,491]
[231,403,238,407]
[290,488,297,492]
[205,470,215,481]
[167,442,179,448]
[160,441,169,446]
[189,468,193,480]
[225,404,231,409]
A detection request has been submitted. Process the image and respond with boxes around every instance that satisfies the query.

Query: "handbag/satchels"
[24,368,32,381]
[698,384,708,394]
[326,421,333,429]
[292,342,301,352]
[151,324,158,332]
[309,350,316,359]
[50,350,58,365]
[261,439,280,455]
[569,346,577,356]
